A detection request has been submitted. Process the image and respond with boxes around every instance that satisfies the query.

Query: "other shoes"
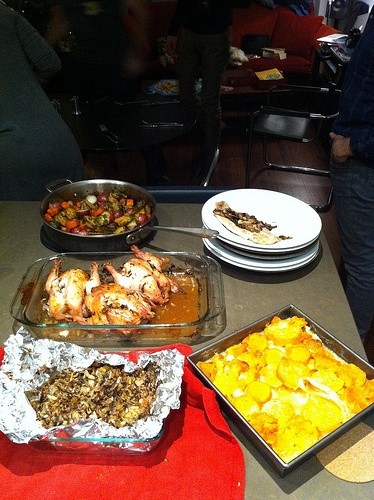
[203,145,219,159]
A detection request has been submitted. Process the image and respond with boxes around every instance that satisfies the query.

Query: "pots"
[40,178,158,248]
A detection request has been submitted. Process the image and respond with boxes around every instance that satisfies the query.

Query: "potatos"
[55,193,151,235]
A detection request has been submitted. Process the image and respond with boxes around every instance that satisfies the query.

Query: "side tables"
[311,43,351,137]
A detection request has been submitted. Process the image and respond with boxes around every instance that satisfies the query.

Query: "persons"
[0,1,85,201]
[166,0,233,162]
[329,5,374,336]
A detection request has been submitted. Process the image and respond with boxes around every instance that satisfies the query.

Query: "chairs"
[51,92,221,187]
[246,84,342,212]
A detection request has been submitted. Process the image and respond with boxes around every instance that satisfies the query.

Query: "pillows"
[272,12,324,58]
[232,7,277,49]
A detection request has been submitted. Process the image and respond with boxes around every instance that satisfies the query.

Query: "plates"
[201,226,321,273]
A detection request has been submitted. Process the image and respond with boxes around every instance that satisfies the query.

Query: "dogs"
[227,45,249,67]
[154,36,179,68]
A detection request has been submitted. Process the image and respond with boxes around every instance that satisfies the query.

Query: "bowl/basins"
[200,189,323,254]
[44,421,165,457]
[9,251,225,347]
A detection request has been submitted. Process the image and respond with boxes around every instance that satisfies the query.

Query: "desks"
[0,202,374,500]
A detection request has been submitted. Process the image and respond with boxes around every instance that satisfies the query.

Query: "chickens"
[102,244,180,305]
[64,282,158,337]
[40,258,102,327]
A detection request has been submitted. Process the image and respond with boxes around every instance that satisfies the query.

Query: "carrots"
[45,194,134,228]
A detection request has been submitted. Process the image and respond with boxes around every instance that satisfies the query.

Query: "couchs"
[125,2,342,83]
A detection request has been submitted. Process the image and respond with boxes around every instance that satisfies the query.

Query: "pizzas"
[213,200,279,244]
